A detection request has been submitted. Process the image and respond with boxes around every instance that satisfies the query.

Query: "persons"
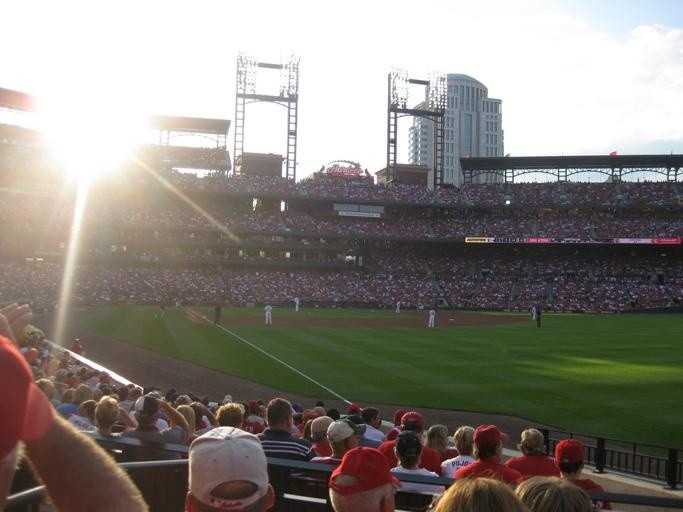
[264,304,273,325]
[393,408,409,426]
[302,419,315,442]
[292,402,304,412]
[379,411,442,475]
[187,402,217,430]
[428,309,436,328]
[327,447,403,512]
[348,416,363,424]
[430,478,524,512]
[175,395,193,407]
[57,384,91,418]
[248,400,260,415]
[441,427,477,476]
[123,396,188,446]
[160,405,196,444]
[61,388,77,402]
[305,409,319,423]
[531,305,537,320]
[87,396,137,443]
[311,417,336,456]
[257,398,314,461]
[314,401,327,417]
[326,419,366,460]
[359,408,384,443]
[515,476,594,512]
[293,413,304,432]
[347,403,361,417]
[385,431,446,498]
[395,301,401,314]
[327,409,340,421]
[0,303,149,511]
[19,345,149,396]
[553,439,601,505]
[235,400,265,434]
[216,403,242,428]
[423,423,459,461]
[387,426,404,442]
[453,425,524,488]
[504,428,561,478]
[215,305,221,324]
[70,400,95,434]
[294,297,299,312]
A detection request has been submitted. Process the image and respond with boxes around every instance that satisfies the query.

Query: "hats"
[0,335,52,462]
[310,415,333,440]
[555,438,585,464]
[349,403,361,416]
[402,410,425,427]
[329,446,404,496]
[305,411,321,419]
[326,419,367,444]
[379,438,400,467]
[397,429,422,466]
[473,424,510,449]
[134,396,158,410]
